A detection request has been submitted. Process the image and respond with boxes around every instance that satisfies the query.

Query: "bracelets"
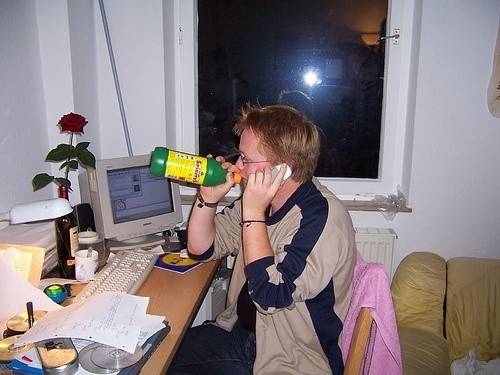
[239,220,267,227]
[196,191,219,209]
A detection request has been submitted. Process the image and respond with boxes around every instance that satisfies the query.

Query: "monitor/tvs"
[78,155,184,251]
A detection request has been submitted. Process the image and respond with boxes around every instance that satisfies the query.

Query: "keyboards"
[73,249,159,304]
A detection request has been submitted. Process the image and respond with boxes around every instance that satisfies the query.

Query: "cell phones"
[270,164,292,185]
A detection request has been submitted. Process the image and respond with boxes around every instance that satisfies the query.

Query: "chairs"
[340,263,387,375]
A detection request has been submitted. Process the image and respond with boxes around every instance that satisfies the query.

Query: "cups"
[78,231,106,267]
[74,249,98,282]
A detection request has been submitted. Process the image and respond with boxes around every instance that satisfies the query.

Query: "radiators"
[354,226,399,280]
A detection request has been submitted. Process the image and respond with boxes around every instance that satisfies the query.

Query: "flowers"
[31,111,97,193]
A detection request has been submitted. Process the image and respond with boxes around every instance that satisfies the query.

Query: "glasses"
[240,152,272,166]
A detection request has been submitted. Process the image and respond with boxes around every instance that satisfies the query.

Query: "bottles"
[150,147,242,187]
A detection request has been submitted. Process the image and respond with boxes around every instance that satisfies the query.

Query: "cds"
[0,310,80,375]
[77,342,143,375]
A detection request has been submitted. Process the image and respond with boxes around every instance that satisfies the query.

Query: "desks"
[59,257,223,375]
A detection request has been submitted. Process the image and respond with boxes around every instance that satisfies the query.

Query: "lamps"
[0,197,74,225]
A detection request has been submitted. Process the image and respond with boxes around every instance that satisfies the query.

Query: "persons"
[164,104,357,375]
[279,45,384,177]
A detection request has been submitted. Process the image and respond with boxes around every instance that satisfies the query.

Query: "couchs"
[392,250,500,375]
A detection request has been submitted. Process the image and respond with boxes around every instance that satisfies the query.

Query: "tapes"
[78,231,99,244]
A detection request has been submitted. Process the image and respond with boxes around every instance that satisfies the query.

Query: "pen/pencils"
[26,301,33,329]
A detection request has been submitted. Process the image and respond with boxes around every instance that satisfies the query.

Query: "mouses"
[179,248,189,259]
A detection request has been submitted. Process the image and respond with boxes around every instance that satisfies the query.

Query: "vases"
[54,186,80,280]
